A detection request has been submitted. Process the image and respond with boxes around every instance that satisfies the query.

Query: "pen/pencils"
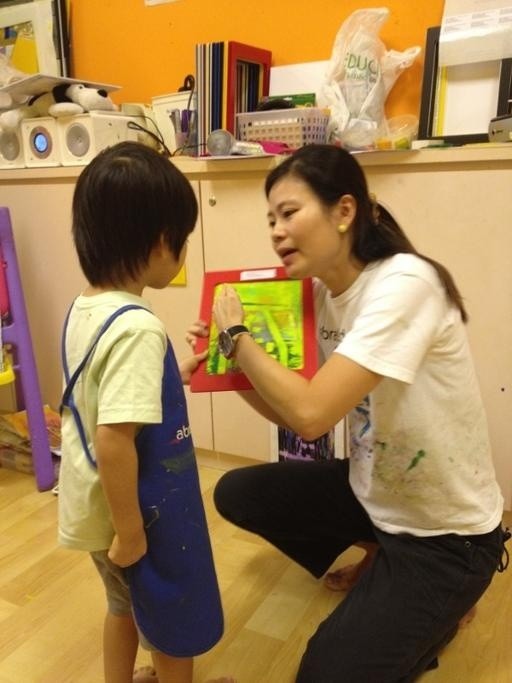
[169,108,196,132]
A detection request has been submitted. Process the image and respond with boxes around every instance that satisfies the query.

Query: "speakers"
[0,111,163,172]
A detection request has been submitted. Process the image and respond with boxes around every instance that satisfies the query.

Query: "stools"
[1,205,55,492]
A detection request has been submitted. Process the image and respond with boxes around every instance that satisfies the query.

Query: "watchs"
[216,325,254,358]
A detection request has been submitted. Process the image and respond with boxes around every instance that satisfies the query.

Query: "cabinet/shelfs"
[1,156,215,469]
[359,144,512,515]
[199,152,359,476]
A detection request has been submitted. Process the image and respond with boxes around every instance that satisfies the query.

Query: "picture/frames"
[190,265,318,393]
[417,22,512,149]
[0,0,71,81]
[272,407,347,464]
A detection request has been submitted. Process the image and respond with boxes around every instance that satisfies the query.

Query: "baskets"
[237,107,329,150]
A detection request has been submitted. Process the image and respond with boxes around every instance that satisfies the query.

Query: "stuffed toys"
[2,82,118,137]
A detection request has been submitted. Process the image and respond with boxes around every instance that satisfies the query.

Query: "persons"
[184,144,508,682]
[58,142,224,682]
